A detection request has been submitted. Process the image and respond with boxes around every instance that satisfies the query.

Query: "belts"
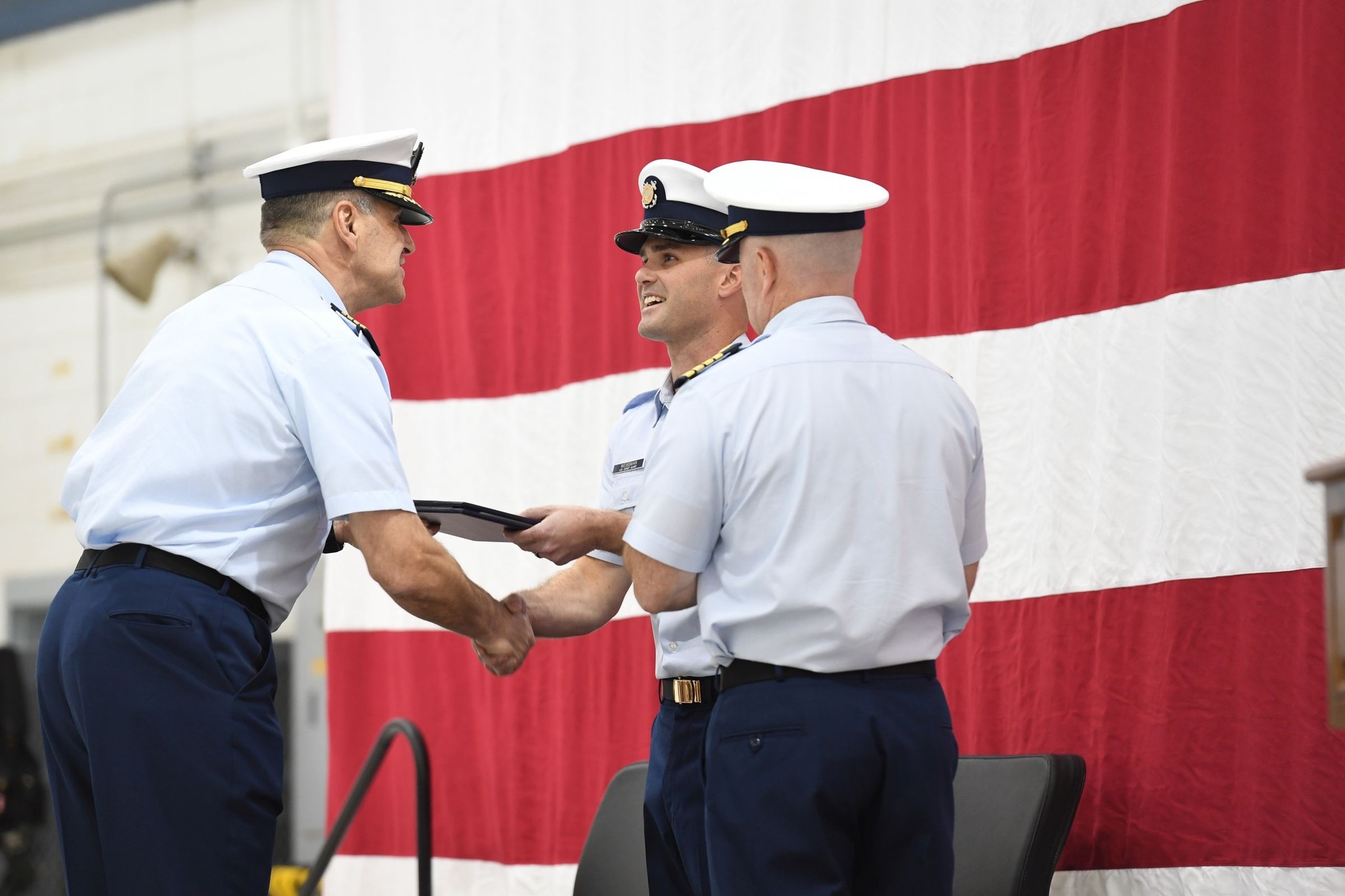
[719,660,937,693]
[74,543,270,622]
[662,679,714,705]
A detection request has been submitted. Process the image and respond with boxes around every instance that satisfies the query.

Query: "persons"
[620,159,986,895]
[472,159,751,896]
[35,131,537,895]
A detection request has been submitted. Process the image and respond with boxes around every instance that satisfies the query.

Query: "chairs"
[572,754,1086,896]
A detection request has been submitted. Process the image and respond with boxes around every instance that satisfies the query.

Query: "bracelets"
[322,521,344,554]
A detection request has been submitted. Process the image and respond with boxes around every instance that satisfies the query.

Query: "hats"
[703,159,890,264]
[243,129,435,226]
[614,158,729,255]
